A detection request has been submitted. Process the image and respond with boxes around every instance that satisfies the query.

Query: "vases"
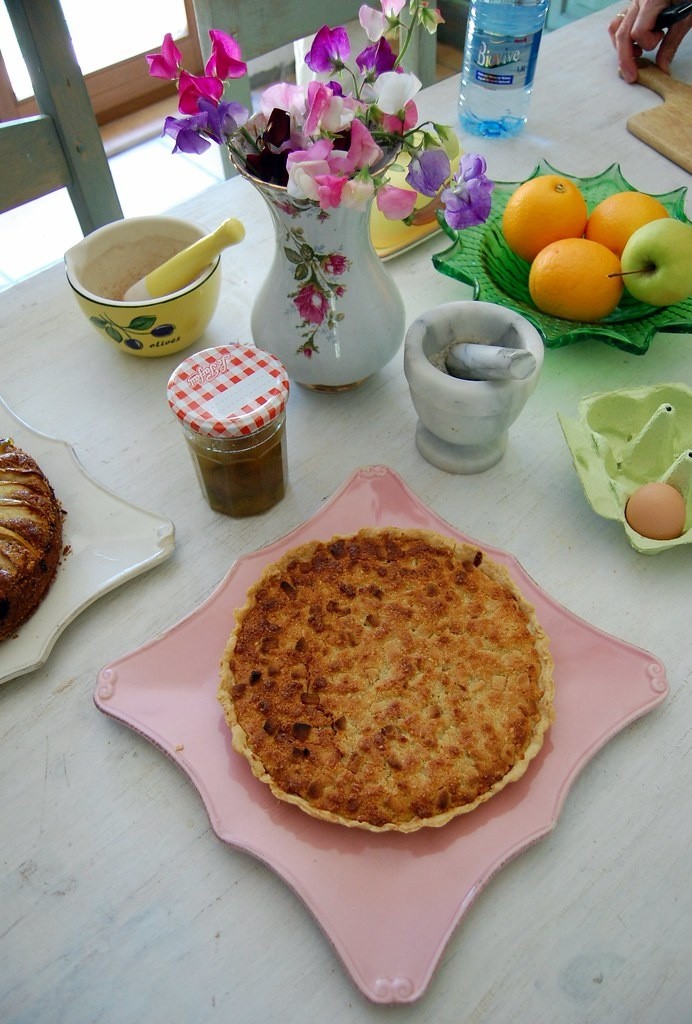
[227,111,407,393]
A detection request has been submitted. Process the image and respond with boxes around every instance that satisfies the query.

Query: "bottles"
[167,344,290,518]
[458,0,549,138]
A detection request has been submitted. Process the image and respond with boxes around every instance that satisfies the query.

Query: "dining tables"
[0,0,692,1024]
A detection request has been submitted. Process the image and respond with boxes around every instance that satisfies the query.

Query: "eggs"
[625,481,686,540]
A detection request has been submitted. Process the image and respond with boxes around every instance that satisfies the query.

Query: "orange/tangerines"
[503,175,587,263]
[585,192,669,259]
[529,238,623,323]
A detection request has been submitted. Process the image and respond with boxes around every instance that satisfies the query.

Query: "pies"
[220,527,556,834]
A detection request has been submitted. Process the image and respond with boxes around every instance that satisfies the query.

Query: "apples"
[607,217,692,306]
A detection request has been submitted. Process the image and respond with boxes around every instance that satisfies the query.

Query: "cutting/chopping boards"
[618,57,692,174]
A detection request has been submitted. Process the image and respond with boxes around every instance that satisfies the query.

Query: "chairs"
[191,0,438,181]
[0,0,124,239]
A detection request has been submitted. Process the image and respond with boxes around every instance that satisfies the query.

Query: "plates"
[96,463,668,1004]
[431,157,692,355]
[0,396,177,685]
[371,129,465,264]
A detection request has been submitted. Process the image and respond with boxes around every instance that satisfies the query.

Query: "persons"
[607,0,692,84]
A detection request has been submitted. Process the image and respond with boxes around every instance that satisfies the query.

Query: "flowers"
[145,0,497,229]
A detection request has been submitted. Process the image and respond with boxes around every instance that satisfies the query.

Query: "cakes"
[0,436,64,639]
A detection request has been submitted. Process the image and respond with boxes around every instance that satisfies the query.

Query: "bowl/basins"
[404,302,544,472]
[64,216,221,358]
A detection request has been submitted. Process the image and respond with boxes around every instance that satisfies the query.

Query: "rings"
[617,13,626,18]
